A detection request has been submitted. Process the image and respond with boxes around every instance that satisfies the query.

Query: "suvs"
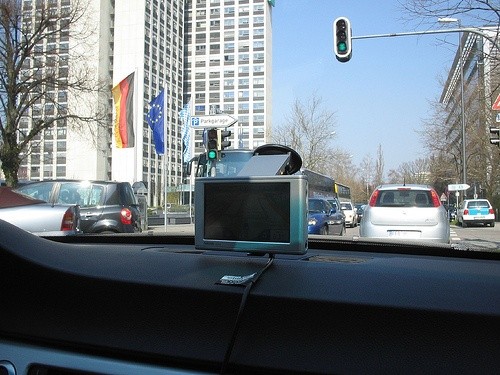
[0,176,147,236]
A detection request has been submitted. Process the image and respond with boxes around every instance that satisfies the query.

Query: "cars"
[307,197,336,235]
[456,199,495,228]
[447,206,457,222]
[358,183,450,245]
[332,201,366,228]
[325,197,347,237]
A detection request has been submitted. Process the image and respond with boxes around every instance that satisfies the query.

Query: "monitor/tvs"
[195,174,310,255]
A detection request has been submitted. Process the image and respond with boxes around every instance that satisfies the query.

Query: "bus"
[292,168,352,203]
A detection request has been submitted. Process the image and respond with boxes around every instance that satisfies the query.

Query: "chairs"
[415,194,427,205]
[383,192,396,205]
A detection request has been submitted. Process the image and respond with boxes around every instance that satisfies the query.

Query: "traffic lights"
[489,128,500,148]
[333,17,352,58]
[205,128,218,161]
[216,129,232,152]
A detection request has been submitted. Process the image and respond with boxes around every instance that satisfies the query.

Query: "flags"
[112,72,136,149]
[177,99,194,162]
[147,91,164,154]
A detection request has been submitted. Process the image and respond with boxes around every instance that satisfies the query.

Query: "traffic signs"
[189,113,239,128]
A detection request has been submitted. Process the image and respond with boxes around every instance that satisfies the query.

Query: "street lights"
[438,16,468,199]
